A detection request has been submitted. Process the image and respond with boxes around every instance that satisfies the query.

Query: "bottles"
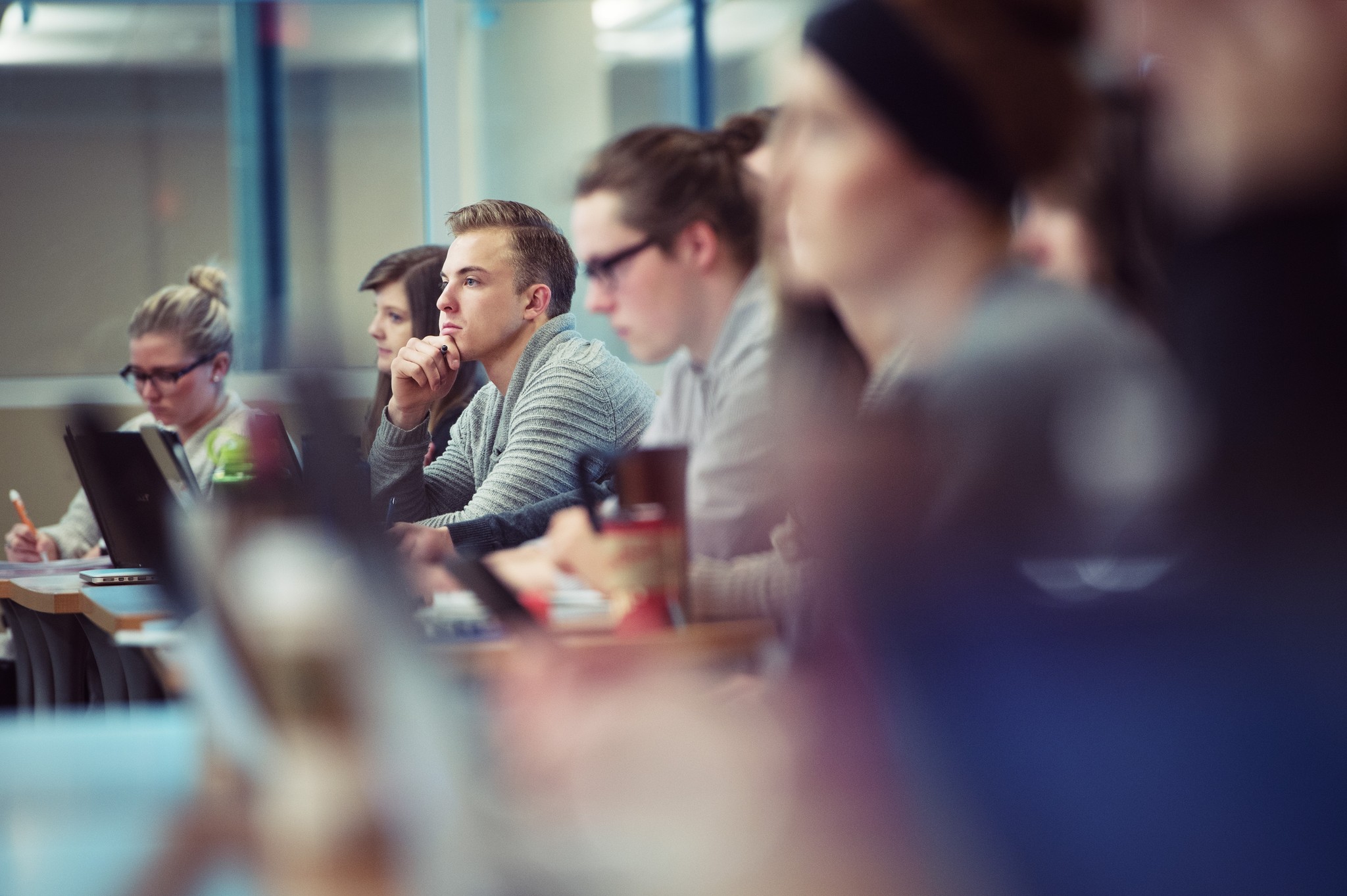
[206,428,252,503]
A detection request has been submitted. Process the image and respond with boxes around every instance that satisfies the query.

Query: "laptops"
[64,424,206,583]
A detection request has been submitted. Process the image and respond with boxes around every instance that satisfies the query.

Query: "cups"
[572,442,692,624]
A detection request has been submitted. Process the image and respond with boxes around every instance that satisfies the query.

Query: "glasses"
[581,237,664,281]
[119,354,216,387]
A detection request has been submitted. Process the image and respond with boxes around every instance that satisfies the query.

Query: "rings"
[6,535,17,548]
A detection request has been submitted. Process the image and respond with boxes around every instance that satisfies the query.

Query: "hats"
[802,1,1097,221]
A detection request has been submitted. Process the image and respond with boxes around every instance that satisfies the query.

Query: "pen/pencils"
[441,345,447,353]
[383,496,397,529]
[7,486,47,562]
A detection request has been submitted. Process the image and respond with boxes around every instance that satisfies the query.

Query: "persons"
[4,265,267,570]
[351,0,1347,896]
